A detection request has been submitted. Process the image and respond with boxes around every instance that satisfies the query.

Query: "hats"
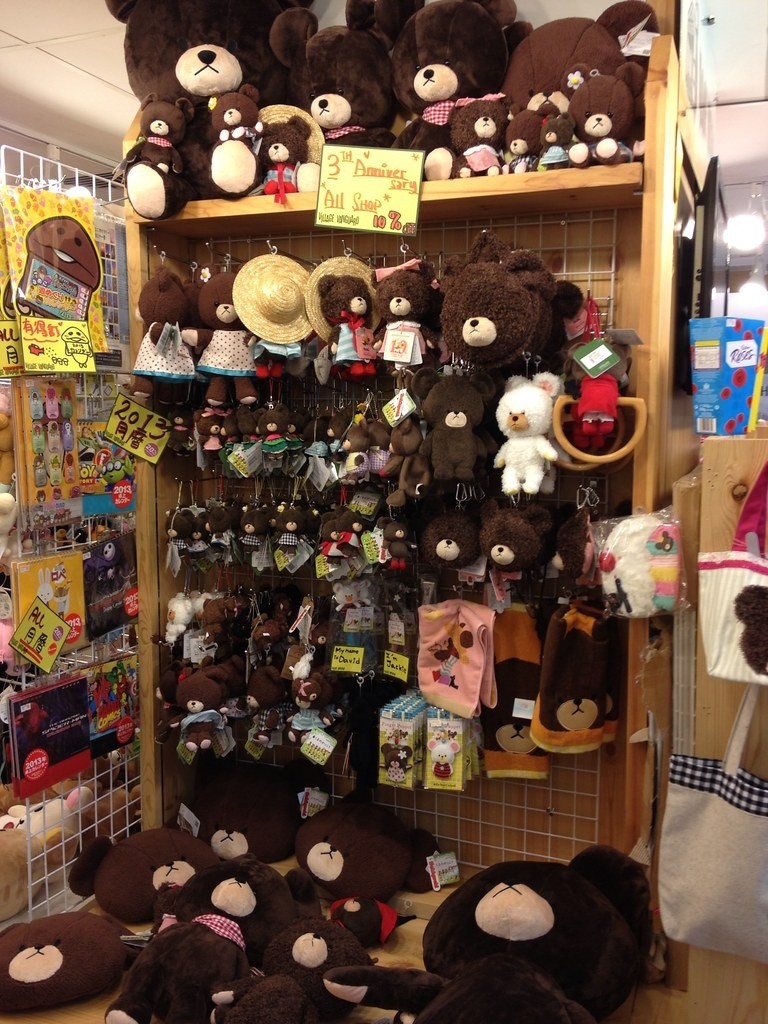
[599,512,681,619]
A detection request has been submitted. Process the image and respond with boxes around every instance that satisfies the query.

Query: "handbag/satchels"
[657,687,767,964]
[698,460,768,686]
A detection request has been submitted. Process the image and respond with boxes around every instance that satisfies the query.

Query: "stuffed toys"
[0,230,680,1024]
[105,0,659,221]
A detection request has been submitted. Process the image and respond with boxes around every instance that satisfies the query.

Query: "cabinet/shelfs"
[119,33,711,992]
